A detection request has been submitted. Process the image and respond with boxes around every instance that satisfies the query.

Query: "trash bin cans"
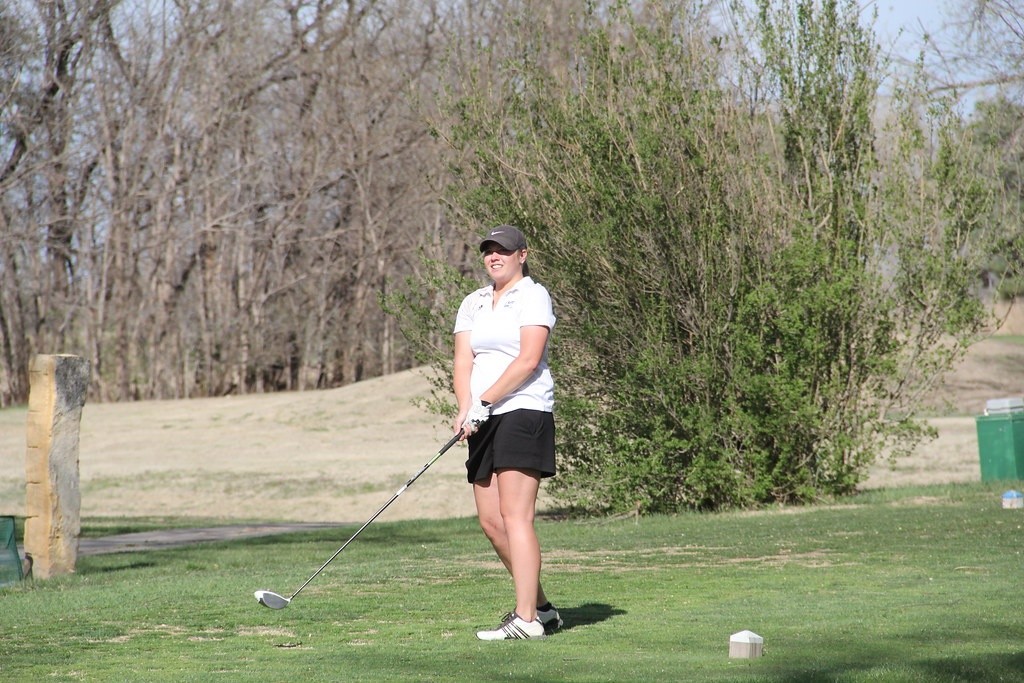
[976,412,1024,481]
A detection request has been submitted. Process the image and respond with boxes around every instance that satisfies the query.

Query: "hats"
[478,225,525,253]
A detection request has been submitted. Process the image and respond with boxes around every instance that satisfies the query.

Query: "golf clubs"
[254,427,464,610]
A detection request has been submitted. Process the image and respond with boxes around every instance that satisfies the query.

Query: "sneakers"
[506,605,564,632]
[474,610,547,641]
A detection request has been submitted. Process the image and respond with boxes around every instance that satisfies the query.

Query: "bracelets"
[481,400,492,409]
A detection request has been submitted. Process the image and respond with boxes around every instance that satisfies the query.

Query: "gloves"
[461,400,492,427]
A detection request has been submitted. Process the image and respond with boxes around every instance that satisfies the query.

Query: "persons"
[452,225,562,640]
